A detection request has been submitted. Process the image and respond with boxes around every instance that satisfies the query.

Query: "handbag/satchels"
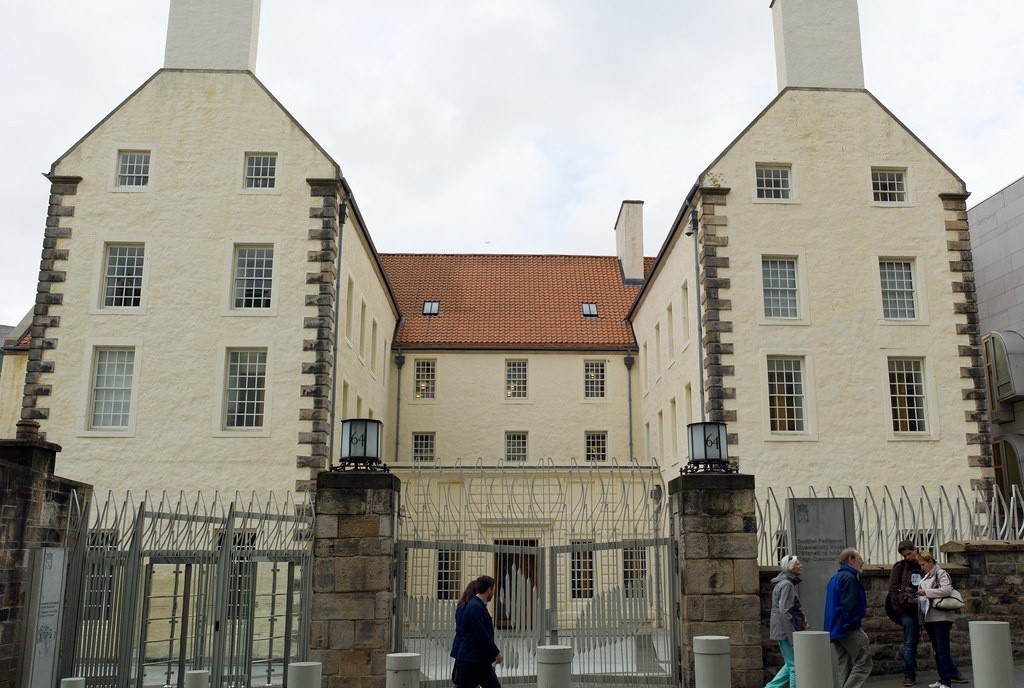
[931,569,965,610]
[885,560,904,626]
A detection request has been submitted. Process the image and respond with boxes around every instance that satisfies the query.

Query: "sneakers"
[940,684,952,688]
[903,676,917,685]
[950,673,969,683]
[928,681,941,688]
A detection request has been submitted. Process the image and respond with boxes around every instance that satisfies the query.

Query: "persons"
[451,576,504,688]
[764,555,810,688]
[905,550,955,688]
[887,540,969,685]
[824,548,873,688]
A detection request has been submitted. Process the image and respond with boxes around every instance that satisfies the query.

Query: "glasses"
[851,556,862,562]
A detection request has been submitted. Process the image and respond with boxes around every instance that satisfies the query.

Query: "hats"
[780,555,797,573]
